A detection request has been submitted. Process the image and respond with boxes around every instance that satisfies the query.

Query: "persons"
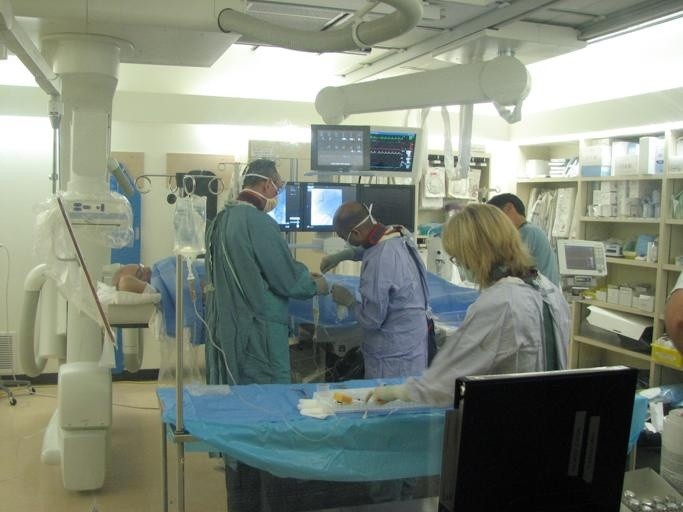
[484,191,562,288]
[114,262,161,296]
[202,160,331,387]
[362,201,572,405]
[660,269,683,361]
[318,199,439,381]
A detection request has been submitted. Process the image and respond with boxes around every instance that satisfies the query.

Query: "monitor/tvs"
[444,363,636,512]
[264,124,425,235]
[555,238,608,277]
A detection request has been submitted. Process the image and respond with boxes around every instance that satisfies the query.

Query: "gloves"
[320,250,351,274]
[312,273,329,295]
[365,385,398,404]
[331,283,355,308]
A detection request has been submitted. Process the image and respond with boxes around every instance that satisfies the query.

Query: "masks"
[456,265,479,283]
[263,195,278,212]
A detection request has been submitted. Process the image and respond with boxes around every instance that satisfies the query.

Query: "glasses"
[449,256,457,265]
[136,263,145,279]
